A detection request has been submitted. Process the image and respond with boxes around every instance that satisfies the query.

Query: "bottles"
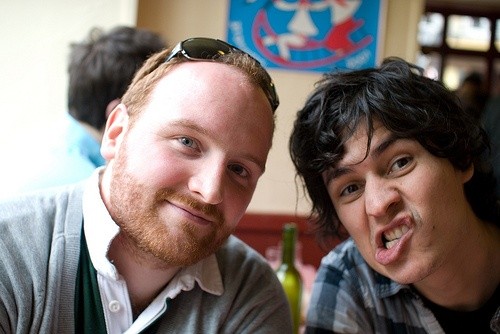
[275,223,302,334]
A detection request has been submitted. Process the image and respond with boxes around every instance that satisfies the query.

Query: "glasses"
[146,37,278,108]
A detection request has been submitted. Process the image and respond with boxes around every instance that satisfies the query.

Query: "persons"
[287,57,500,334]
[0,39,293,334]
[64,24,167,184]
[451,72,487,120]
